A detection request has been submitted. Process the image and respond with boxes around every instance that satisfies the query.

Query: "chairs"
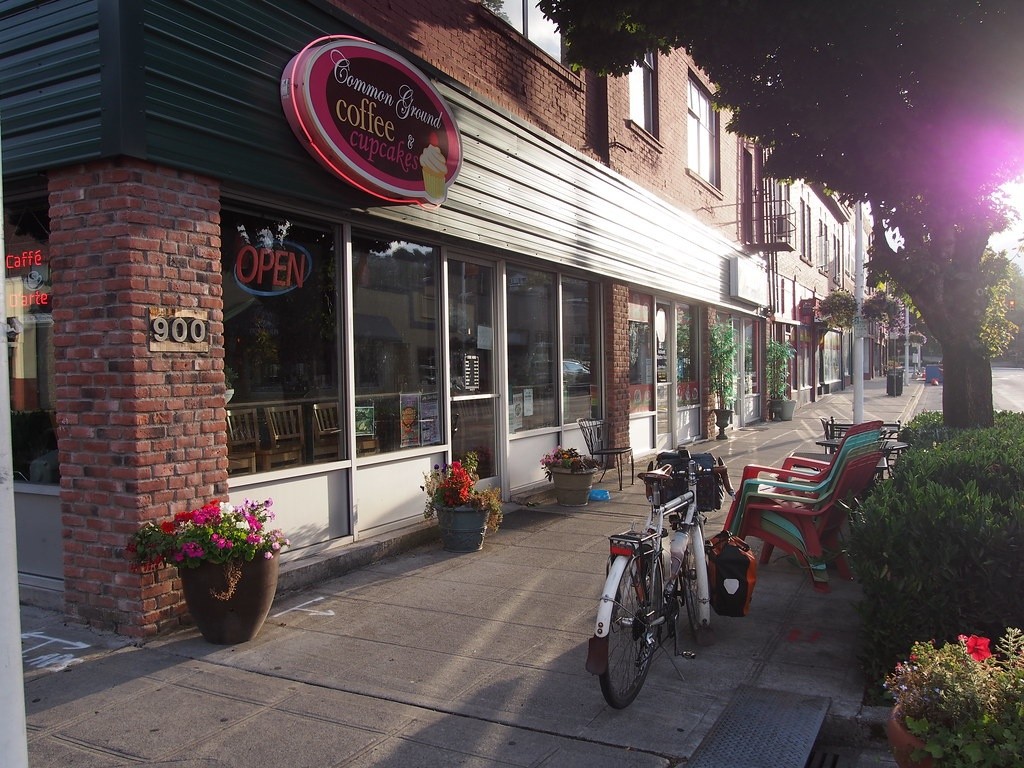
[226,408,260,475]
[356,401,384,457]
[312,400,344,459]
[578,418,635,491]
[261,406,303,471]
[722,408,906,588]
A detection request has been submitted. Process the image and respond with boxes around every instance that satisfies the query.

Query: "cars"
[447,376,484,436]
[530,358,591,395]
[419,365,437,383]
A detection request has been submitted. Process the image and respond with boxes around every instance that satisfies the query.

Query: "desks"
[819,416,901,454]
[812,438,911,479]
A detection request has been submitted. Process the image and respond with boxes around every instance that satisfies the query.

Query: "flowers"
[128,494,292,571]
[424,452,504,516]
[886,624,1023,738]
[539,449,590,478]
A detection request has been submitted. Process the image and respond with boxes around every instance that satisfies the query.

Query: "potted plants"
[710,323,738,440]
[766,340,794,421]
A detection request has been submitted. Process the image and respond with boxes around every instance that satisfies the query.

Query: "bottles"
[661,528,671,580]
[670,523,690,578]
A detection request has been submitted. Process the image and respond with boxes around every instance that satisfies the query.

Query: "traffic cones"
[922,369,926,378]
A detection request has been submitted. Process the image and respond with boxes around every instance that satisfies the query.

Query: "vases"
[887,684,1016,767]
[178,539,287,646]
[547,466,596,508]
[436,502,493,551]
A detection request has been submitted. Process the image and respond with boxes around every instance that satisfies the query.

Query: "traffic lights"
[1009,300,1015,312]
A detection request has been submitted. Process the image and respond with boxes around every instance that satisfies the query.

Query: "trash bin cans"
[925,365,944,383]
[886,370,903,396]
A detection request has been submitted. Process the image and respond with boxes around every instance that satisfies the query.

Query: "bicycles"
[585,457,733,710]
